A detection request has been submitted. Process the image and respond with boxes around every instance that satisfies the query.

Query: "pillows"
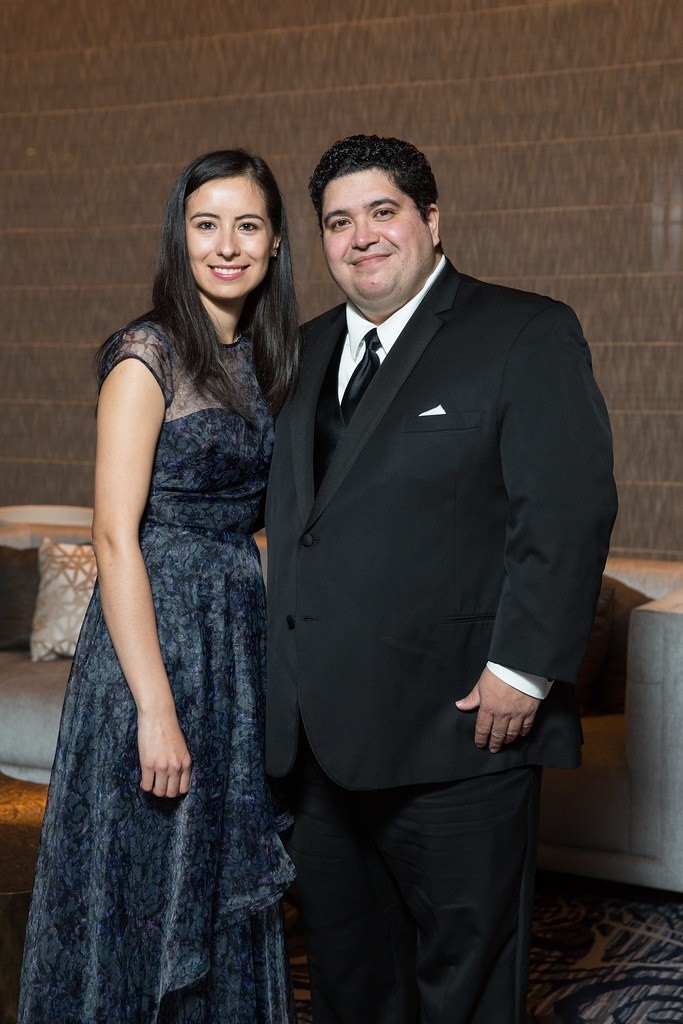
[29,536,97,663]
[0,544,40,652]
[575,575,655,718]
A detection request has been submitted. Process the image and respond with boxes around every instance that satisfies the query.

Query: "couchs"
[0,505,683,906]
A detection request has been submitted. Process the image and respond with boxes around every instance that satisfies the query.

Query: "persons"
[21,147,298,1024]
[264,139,621,1024]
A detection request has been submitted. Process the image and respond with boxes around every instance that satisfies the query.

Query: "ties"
[340,328,382,426]
